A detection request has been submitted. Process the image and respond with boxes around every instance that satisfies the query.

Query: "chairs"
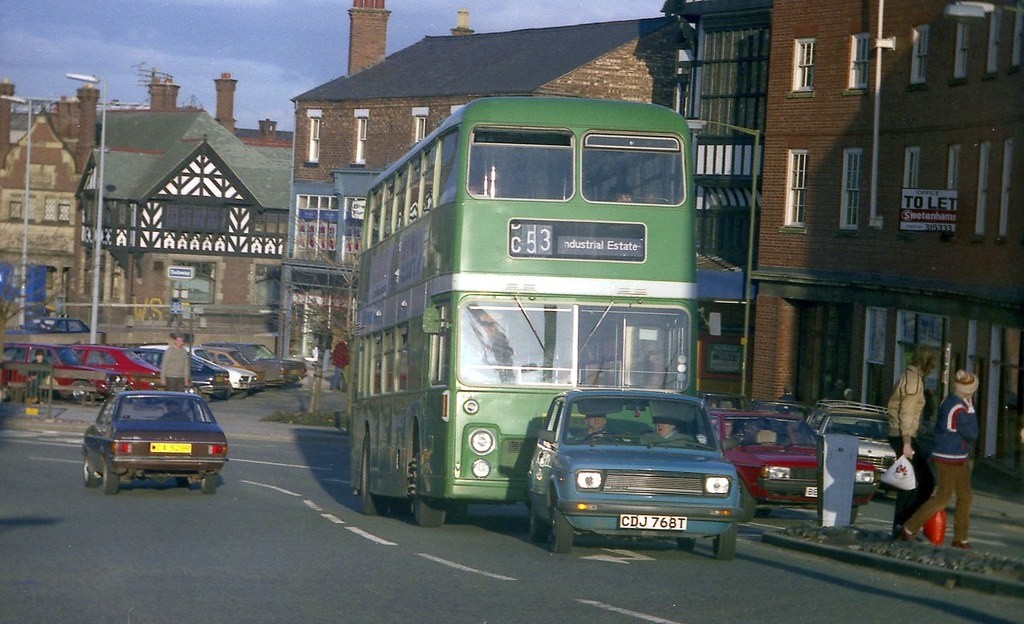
[98,352,106,365]
[374,373,408,392]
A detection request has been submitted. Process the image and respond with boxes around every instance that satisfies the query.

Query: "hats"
[954,370,978,394]
[911,345,937,374]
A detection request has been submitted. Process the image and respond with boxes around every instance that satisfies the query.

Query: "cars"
[3,317,90,333]
[81,390,230,495]
[58,342,309,401]
[696,390,900,522]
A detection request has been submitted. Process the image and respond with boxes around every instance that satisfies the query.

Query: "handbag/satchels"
[880,450,916,490]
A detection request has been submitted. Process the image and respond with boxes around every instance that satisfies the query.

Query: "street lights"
[66,72,109,345]
[0,93,34,328]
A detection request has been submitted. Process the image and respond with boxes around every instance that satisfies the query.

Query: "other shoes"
[950,540,971,549]
[898,527,922,543]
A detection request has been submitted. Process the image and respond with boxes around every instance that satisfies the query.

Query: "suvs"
[0,341,135,406]
[526,387,744,558]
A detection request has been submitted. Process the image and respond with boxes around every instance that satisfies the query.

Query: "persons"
[613,183,633,202]
[829,379,853,401]
[641,417,694,445]
[331,339,351,390]
[898,369,979,549]
[887,346,937,537]
[157,400,191,421]
[573,414,615,440]
[439,171,481,202]
[715,420,744,443]
[161,334,192,406]
[776,387,795,413]
[26,349,50,405]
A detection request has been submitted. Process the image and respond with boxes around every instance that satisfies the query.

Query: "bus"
[346,95,701,528]
